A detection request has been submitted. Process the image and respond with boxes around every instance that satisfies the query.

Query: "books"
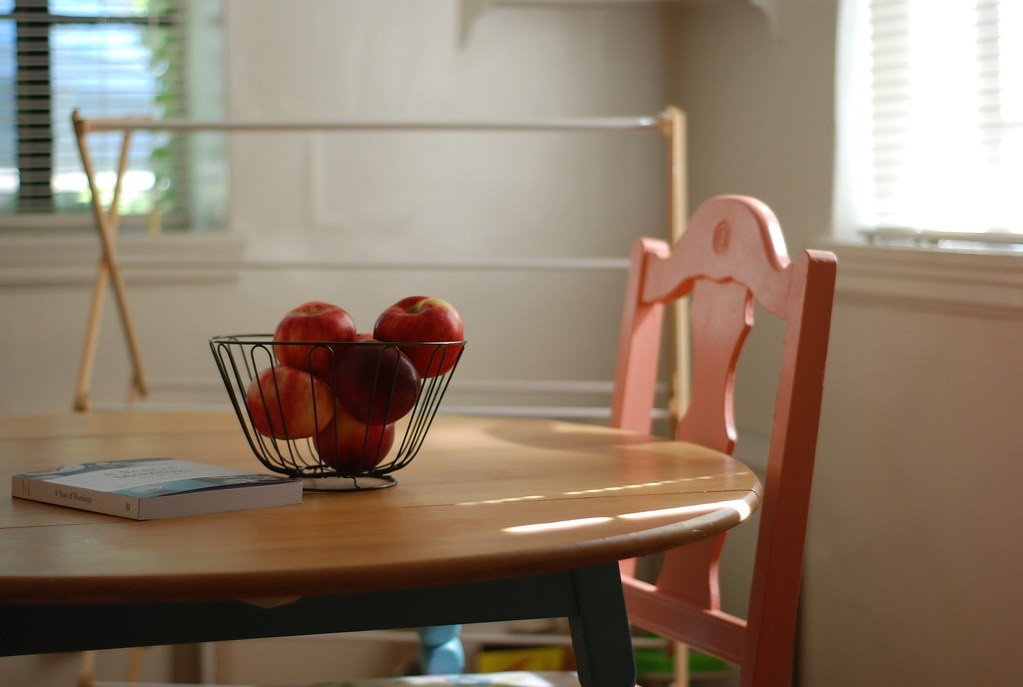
[11,457,304,520]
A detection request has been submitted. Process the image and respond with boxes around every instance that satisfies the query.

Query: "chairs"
[352,193,837,687]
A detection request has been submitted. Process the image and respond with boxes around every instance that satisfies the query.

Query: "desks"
[0,410,763,687]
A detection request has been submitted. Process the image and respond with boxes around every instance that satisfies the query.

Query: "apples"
[246,296,464,472]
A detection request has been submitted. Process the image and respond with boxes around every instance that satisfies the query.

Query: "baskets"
[209,334,468,492]
